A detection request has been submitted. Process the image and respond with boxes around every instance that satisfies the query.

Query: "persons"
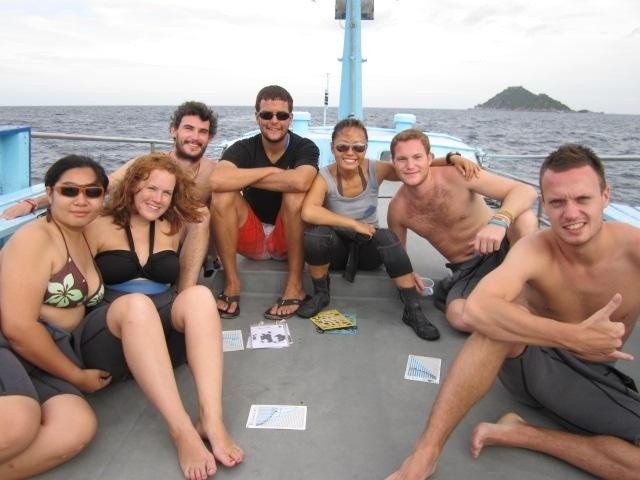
[71,154,244,480]
[210,85,320,320]
[0,154,108,480]
[0,102,218,276]
[387,129,539,333]
[383,143,640,480]
[296,118,481,339]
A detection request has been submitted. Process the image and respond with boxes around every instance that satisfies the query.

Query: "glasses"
[333,143,366,153]
[257,111,291,121]
[51,185,105,198]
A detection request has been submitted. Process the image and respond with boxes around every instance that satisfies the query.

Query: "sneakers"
[296,289,332,318]
[402,304,440,341]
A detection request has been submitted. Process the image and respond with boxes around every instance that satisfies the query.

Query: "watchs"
[446,151,461,166]
[24,199,38,213]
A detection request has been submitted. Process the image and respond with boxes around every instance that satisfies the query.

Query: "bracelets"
[487,209,514,230]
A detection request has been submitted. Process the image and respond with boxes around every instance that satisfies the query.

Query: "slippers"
[215,290,241,320]
[263,292,312,321]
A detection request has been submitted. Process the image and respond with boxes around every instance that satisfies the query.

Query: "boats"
[0,0,640,478]
